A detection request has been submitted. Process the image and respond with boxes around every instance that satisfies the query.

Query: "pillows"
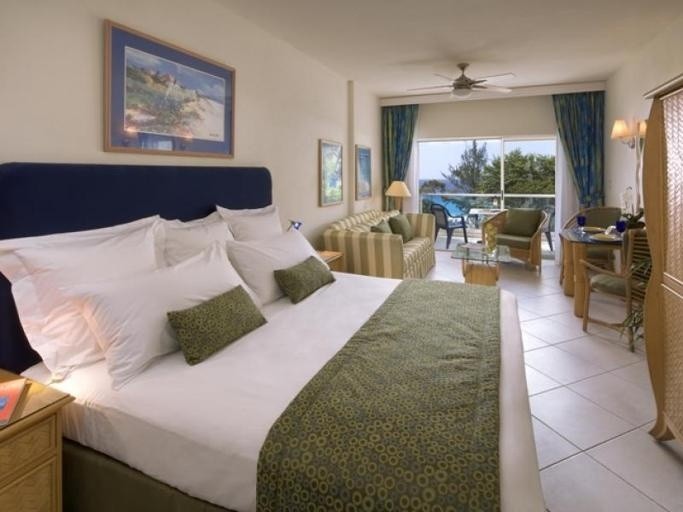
[346,210,414,244]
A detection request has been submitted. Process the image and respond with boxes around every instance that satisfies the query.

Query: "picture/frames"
[318,138,344,207]
[354,143,373,201]
[102,18,237,160]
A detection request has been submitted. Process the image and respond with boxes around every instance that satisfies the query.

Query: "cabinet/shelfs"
[641,72,683,449]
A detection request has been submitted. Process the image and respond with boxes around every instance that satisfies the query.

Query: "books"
[0,377,27,426]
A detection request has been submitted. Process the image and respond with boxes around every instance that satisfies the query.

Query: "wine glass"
[576,215,586,234]
[615,220,625,237]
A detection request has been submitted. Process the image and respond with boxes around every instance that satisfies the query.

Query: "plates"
[589,234,623,244]
[581,227,604,233]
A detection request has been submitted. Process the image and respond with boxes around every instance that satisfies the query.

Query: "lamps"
[286,217,303,231]
[384,180,412,215]
[610,119,648,148]
[451,85,472,97]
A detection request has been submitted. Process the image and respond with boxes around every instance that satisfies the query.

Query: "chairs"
[431,203,467,250]
[483,204,651,353]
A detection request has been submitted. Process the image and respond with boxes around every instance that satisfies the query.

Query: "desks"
[469,208,509,243]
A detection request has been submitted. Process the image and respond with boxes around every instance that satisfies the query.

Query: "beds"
[0,163,551,512]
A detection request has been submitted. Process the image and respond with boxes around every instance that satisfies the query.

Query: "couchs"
[323,208,436,280]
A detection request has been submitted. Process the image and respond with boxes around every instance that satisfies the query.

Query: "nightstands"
[0,367,78,512]
[317,251,345,272]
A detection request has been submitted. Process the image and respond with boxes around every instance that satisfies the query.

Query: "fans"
[406,62,514,98]
